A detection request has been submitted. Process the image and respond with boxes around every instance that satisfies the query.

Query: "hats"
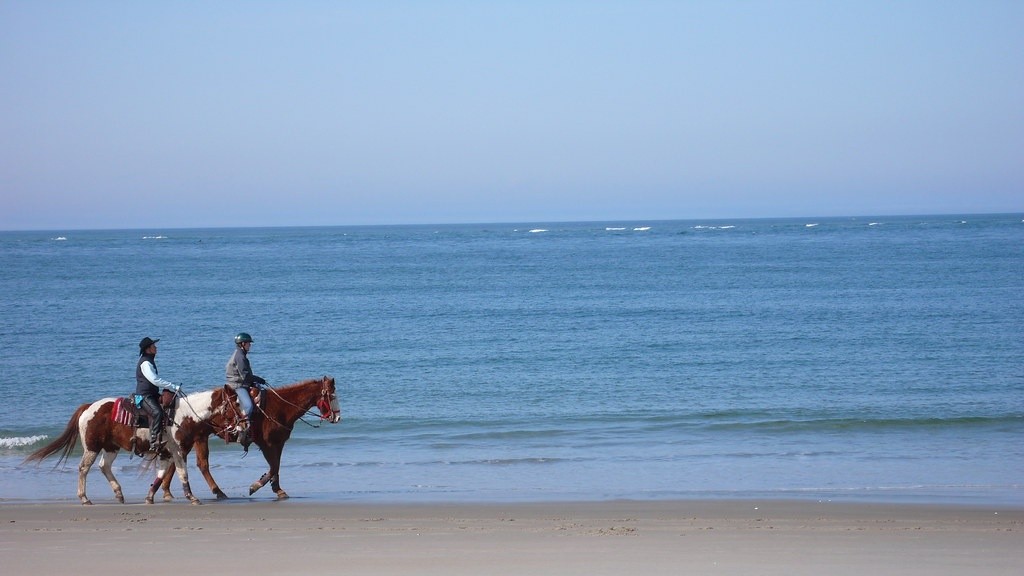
[139,337,160,356]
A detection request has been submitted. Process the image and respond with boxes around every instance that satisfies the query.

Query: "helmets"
[235,333,254,349]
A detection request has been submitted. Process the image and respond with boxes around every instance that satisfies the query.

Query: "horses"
[19,384,251,506]
[162,376,342,499]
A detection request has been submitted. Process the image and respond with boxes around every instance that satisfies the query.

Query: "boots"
[149,432,167,451]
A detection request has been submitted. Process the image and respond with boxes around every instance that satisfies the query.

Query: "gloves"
[171,383,180,391]
[251,375,266,385]
[251,383,256,387]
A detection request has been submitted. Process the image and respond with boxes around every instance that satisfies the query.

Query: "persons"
[136,336,181,451]
[225,333,266,443]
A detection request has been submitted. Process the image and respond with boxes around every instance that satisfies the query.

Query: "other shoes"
[237,433,253,442]
